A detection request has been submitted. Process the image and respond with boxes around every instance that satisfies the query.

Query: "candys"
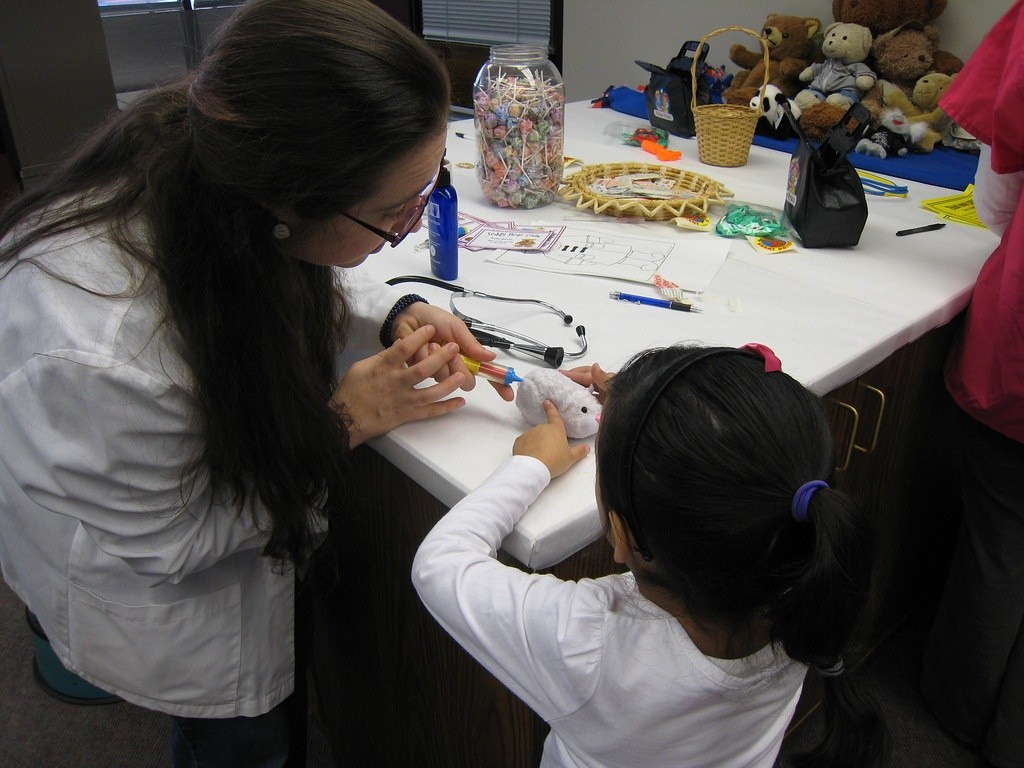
[471,62,565,209]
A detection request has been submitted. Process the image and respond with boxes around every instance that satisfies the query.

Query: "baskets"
[689,25,769,167]
[558,161,736,221]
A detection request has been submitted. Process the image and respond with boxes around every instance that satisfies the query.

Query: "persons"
[410,345,892,768]
[909,0,1024,768]
[1,0,514,768]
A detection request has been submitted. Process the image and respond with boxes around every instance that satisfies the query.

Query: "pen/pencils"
[455,132,475,142]
[781,102,814,153]
[609,291,704,313]
[896,223,948,237]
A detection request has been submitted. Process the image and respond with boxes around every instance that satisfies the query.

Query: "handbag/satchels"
[774,91,872,250]
[635,40,711,139]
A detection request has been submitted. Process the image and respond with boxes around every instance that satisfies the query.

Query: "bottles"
[427,147,459,280]
[473,44,566,211]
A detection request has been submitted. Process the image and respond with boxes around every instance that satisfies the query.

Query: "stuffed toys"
[515,367,603,439]
[722,0,965,159]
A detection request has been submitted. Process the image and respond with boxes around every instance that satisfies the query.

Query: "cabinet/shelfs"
[321,96,1002,768]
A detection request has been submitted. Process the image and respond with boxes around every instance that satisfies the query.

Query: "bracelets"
[379,294,429,349]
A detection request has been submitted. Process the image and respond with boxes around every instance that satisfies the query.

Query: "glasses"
[339,144,448,247]
